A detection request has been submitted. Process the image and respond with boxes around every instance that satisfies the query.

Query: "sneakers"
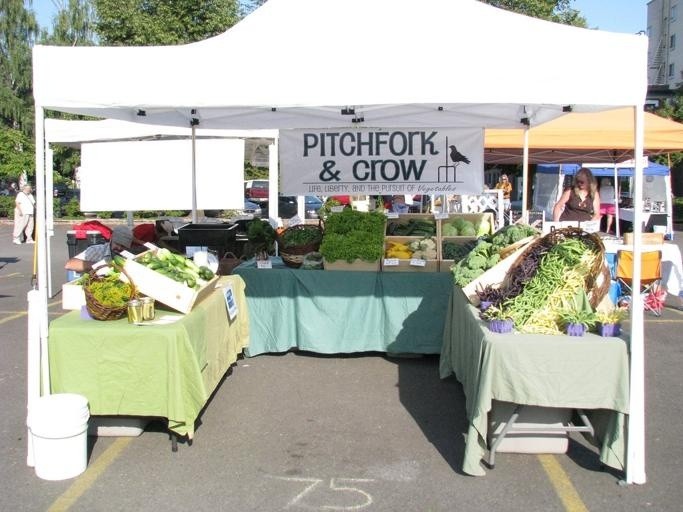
[12,238,22,245]
[25,238,36,244]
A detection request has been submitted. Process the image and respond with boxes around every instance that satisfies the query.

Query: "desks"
[231,253,447,358]
[47,274,252,450]
[439,271,629,470]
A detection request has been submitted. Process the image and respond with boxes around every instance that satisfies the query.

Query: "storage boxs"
[61,274,87,311]
[119,250,221,315]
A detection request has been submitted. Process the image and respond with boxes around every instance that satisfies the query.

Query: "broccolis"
[448,223,536,287]
[410,238,437,259]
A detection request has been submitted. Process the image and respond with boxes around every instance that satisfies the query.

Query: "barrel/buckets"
[26,393,90,482]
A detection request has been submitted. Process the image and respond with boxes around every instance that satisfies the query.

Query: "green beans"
[490,237,628,334]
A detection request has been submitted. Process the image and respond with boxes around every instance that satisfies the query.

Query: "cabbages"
[442,215,491,237]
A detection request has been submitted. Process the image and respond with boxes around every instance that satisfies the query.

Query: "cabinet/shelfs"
[322,211,493,272]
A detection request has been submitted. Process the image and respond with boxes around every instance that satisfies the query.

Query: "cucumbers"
[386,217,435,237]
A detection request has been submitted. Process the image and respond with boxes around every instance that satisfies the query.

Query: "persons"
[20,191,34,241]
[11,181,36,244]
[127,221,174,255]
[63,225,133,273]
[598,177,615,234]
[491,173,512,213]
[552,168,601,222]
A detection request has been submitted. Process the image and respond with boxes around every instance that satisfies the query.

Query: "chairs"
[615,250,662,317]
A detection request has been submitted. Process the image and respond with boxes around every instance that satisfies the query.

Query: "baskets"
[220,224,323,276]
[83,263,135,321]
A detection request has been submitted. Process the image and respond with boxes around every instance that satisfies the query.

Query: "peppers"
[137,252,214,288]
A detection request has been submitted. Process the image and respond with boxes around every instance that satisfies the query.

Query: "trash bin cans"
[66,230,113,260]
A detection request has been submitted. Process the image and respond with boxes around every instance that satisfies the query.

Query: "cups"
[127,297,156,325]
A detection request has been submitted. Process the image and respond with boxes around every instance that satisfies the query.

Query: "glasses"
[576,180,585,185]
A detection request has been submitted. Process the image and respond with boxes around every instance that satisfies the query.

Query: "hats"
[110,224,133,249]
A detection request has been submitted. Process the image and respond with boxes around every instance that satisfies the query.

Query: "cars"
[200,177,332,218]
